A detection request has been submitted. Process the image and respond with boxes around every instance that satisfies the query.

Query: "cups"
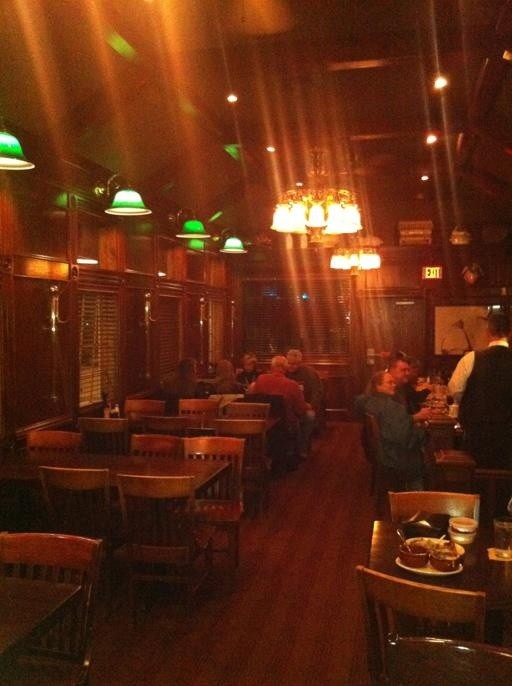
[493,518,511,560]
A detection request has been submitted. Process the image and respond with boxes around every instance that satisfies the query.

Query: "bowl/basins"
[429,544,462,569]
[447,526,478,543]
[398,544,427,568]
[449,516,479,533]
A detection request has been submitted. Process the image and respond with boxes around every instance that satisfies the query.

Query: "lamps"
[1,121,37,175]
[167,205,211,242]
[270,145,365,238]
[208,226,248,256]
[94,170,158,220]
[327,234,384,273]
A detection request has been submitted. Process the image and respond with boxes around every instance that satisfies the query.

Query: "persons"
[163,357,219,415]
[247,354,312,470]
[353,370,452,529]
[380,349,432,422]
[442,310,511,524]
[213,348,324,458]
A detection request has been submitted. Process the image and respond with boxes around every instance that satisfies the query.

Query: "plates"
[402,536,466,553]
[395,557,465,576]
